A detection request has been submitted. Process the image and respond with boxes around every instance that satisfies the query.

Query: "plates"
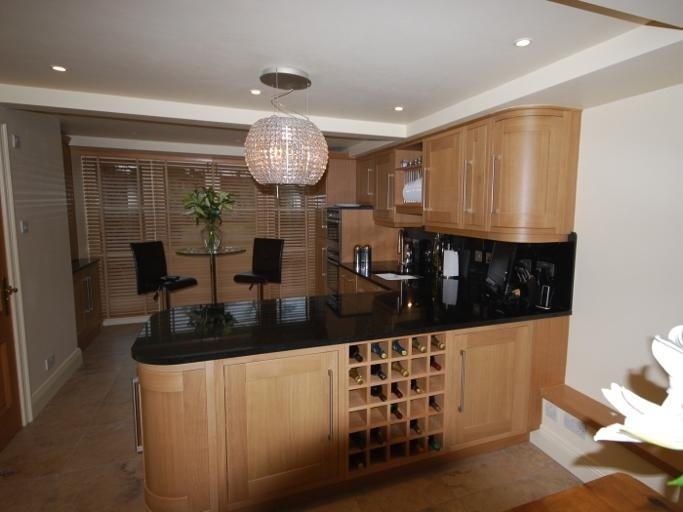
[334,203,363,209]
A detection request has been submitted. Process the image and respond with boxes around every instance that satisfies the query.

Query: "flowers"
[182,186,240,248]
[593,324,683,488]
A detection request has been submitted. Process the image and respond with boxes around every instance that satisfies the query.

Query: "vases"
[201,222,222,251]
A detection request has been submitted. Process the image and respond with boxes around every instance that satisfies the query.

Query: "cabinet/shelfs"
[356,106,582,244]
[131,261,569,511]
[72,257,102,348]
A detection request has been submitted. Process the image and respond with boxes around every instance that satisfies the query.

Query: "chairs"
[233,237,284,299]
[130,240,197,308]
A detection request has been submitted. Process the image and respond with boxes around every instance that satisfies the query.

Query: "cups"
[423,248,431,257]
[443,247,461,277]
[442,277,459,307]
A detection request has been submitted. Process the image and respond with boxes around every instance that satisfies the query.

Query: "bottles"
[432,232,453,270]
[353,244,371,265]
[402,239,416,266]
[403,267,414,287]
[399,158,421,167]
[430,271,446,310]
[344,335,446,470]
[354,262,368,279]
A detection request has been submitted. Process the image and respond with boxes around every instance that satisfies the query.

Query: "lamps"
[243,66,329,186]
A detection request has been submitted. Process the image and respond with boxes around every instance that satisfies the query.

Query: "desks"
[176,246,246,304]
[504,472,682,511]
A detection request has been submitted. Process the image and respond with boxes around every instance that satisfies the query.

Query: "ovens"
[325,207,339,294]
[325,297,341,314]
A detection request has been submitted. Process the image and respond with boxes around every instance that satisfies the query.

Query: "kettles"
[536,279,556,309]
